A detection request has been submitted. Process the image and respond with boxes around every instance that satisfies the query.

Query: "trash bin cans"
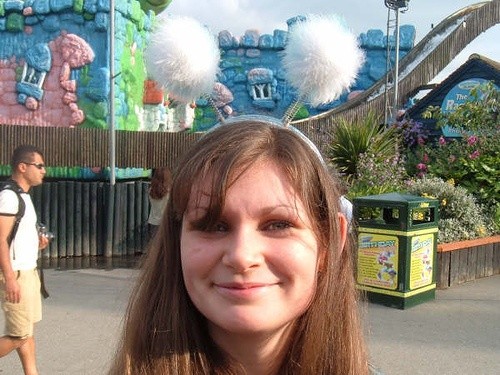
[351,192,436,311]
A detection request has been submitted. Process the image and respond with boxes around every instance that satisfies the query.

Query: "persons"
[146,166,174,242]
[108,115,370,375]
[337,184,353,242]
[0,144,46,375]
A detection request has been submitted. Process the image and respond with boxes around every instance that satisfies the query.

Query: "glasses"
[20,161,44,169]
